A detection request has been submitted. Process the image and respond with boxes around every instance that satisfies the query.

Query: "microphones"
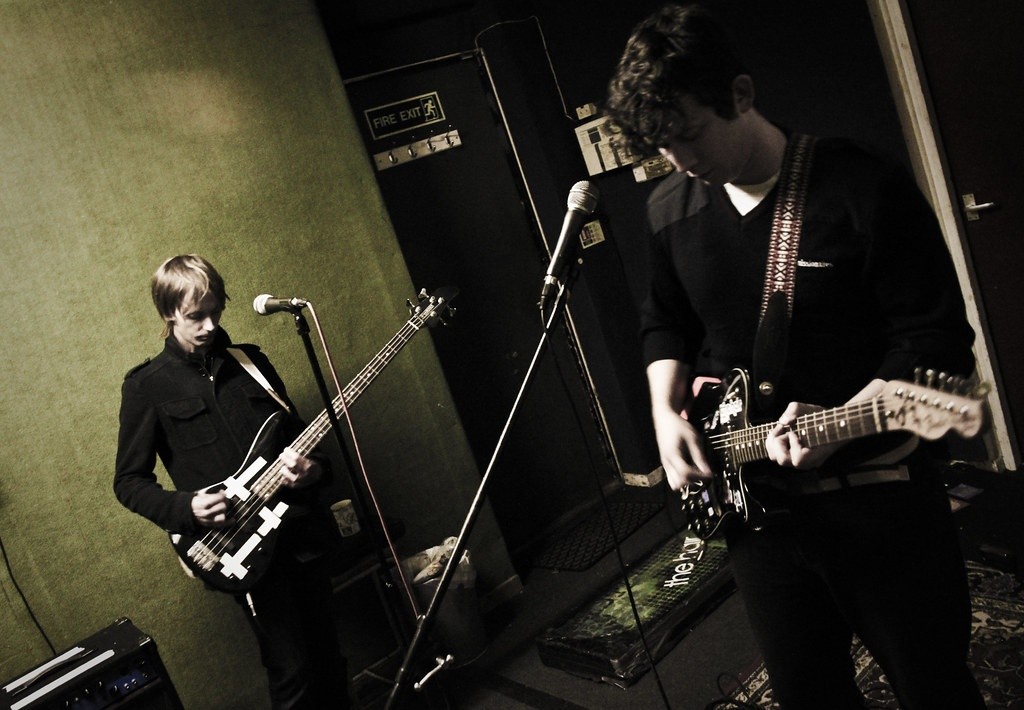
[252,293,309,317]
[540,181,600,311]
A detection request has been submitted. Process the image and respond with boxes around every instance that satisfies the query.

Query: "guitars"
[166,281,461,596]
[674,360,996,545]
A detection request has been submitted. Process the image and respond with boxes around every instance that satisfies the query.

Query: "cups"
[330,499,360,537]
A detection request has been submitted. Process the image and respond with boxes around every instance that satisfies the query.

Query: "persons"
[113,255,353,710]
[606,6,984,710]
